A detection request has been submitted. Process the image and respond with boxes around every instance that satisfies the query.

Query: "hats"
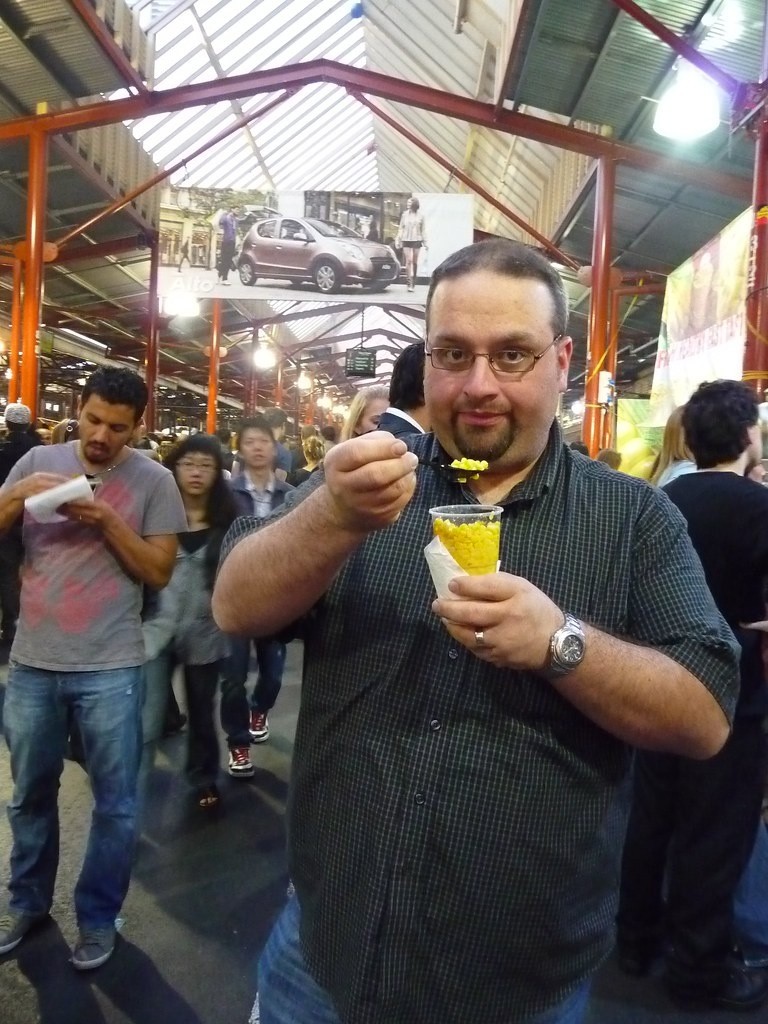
[5,403,30,424]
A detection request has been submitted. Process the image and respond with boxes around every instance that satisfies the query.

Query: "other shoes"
[198,782,221,809]
[221,279,230,285]
[408,287,414,291]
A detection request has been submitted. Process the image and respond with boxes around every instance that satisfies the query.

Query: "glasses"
[424,334,565,373]
[176,462,217,471]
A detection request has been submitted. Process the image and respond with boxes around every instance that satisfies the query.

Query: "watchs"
[542,610,586,680]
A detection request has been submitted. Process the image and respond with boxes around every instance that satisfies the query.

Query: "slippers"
[162,712,186,732]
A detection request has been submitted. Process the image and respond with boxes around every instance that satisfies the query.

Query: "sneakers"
[0,907,49,953]
[249,708,270,743]
[228,747,254,778]
[73,920,116,969]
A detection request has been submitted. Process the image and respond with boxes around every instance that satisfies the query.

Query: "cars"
[233,216,401,295]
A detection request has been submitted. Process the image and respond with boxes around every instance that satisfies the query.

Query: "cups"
[428,504,503,578]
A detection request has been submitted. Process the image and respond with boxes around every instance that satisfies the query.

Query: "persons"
[354,216,362,233]
[140,433,242,809]
[0,404,45,667]
[284,434,301,450]
[211,245,743,1024]
[569,441,623,471]
[377,340,432,438]
[322,426,336,451]
[31,417,81,445]
[618,376,768,1024]
[213,427,234,473]
[291,424,317,468]
[231,407,292,481]
[291,436,326,487]
[340,385,389,436]
[395,197,430,292]
[178,236,190,273]
[222,414,295,778]
[214,205,252,285]
[0,365,190,972]
[366,219,378,241]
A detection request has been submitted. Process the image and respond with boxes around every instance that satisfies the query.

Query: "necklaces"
[77,442,133,476]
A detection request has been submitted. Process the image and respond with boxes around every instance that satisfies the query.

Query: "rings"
[79,514,82,522]
[475,627,484,646]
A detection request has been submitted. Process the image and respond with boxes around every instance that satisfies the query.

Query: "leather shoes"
[662,960,768,1008]
[621,917,663,969]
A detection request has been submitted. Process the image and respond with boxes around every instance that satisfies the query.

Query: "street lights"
[291,346,333,436]
